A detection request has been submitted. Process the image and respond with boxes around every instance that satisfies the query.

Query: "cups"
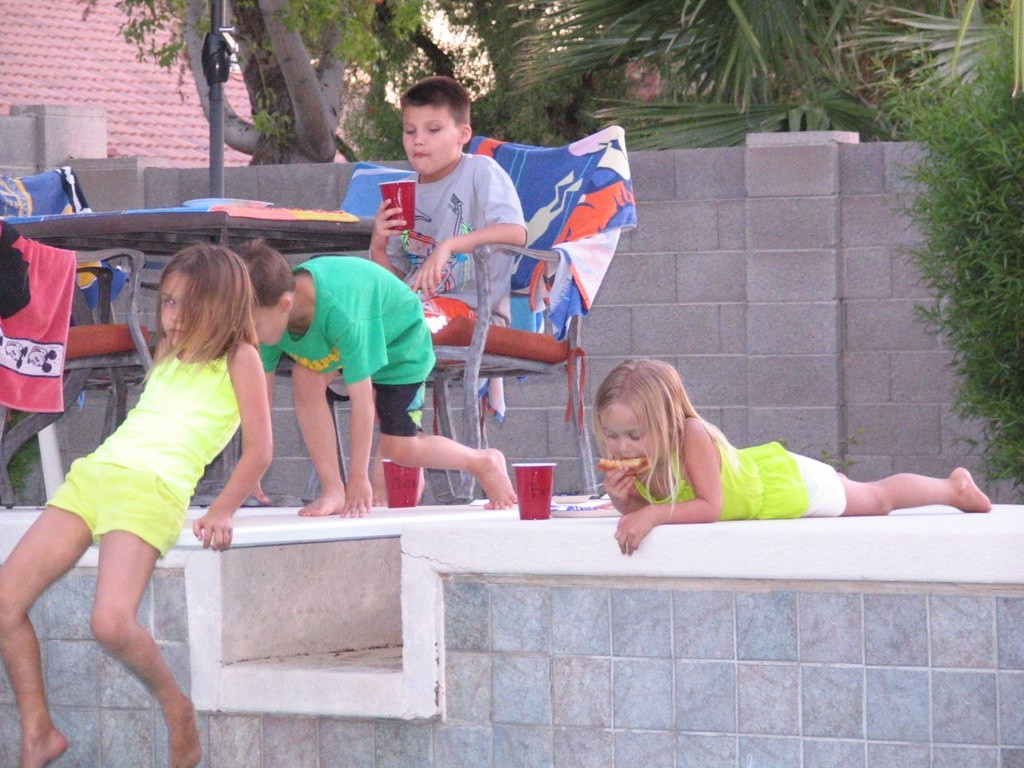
[379,181,416,230]
[383,459,420,508]
[513,464,556,520]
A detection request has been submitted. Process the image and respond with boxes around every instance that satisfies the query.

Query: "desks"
[0,204,377,506]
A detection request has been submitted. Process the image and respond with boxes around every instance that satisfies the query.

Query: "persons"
[369,78,527,508]
[236,241,518,520]
[590,357,992,556]
[0,244,273,768]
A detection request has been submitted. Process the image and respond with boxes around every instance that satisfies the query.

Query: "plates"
[183,198,275,207]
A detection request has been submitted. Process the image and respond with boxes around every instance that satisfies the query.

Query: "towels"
[0,159,130,413]
[343,124,637,426]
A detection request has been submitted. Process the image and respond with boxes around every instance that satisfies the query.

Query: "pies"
[599,458,651,474]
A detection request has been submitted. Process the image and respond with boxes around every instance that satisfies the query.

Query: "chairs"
[301,126,638,505]
[0,167,150,508]
